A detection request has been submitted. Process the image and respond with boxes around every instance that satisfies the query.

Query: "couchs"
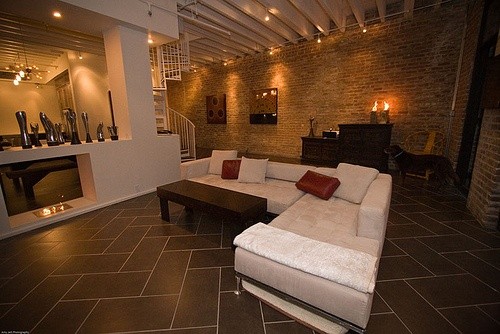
[179,156,393,334]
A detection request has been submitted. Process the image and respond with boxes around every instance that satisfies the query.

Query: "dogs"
[384,143,454,193]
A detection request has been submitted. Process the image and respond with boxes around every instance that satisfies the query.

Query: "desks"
[5,159,74,200]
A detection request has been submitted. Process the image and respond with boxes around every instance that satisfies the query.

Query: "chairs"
[401,129,445,180]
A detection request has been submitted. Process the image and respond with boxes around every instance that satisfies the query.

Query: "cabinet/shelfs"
[300,136,340,168]
[339,122,393,171]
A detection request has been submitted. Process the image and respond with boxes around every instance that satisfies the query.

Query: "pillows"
[237,155,268,183]
[210,149,237,175]
[295,169,340,197]
[331,162,379,205]
[221,159,242,177]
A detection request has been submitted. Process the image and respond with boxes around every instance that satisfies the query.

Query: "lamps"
[5,46,52,82]
[7,52,26,87]
